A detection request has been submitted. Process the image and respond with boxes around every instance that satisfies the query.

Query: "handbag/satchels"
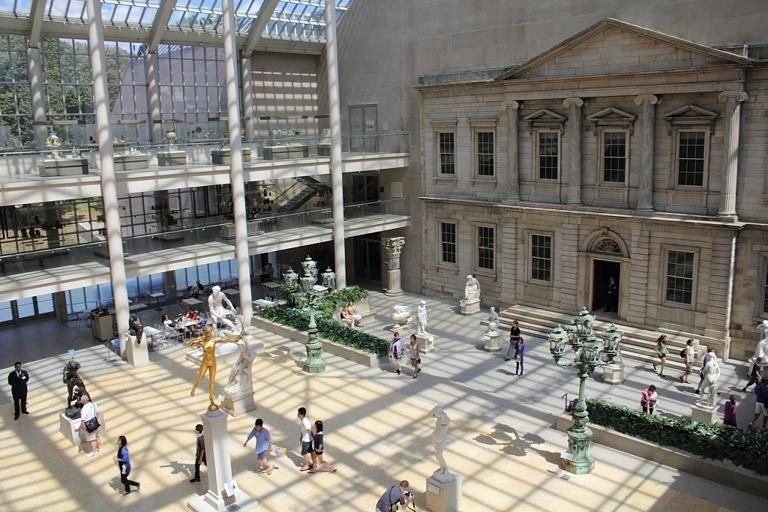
[84,417,101,433]
[680,349,686,358]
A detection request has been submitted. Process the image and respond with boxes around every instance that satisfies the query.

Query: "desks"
[93,280,282,363]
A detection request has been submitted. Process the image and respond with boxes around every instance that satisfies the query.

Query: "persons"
[429,405,451,476]
[503,320,521,362]
[640,320,768,433]
[605,275,619,315]
[487,306,500,333]
[340,306,353,326]
[415,299,428,338]
[514,337,525,376]
[347,300,365,326]
[266,262,274,274]
[296,407,336,474]
[189,424,207,483]
[63,361,102,452]
[208,284,237,328]
[375,480,415,512]
[462,274,480,299]
[409,334,421,380]
[389,332,406,376]
[163,311,207,342]
[243,418,274,472]
[8,361,29,420]
[130,311,152,348]
[113,435,140,494]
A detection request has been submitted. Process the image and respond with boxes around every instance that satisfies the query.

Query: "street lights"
[547,306,624,474]
[281,254,337,373]
